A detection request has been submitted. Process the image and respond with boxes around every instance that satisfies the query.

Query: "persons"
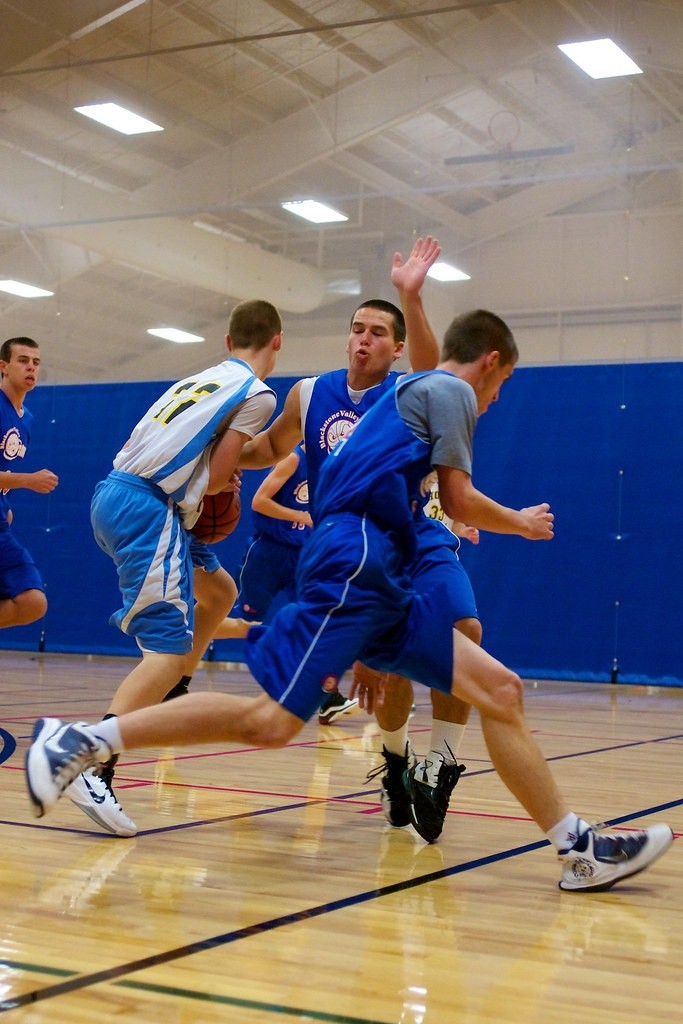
[0,337,59,630]
[34,728,668,1023]
[25,309,673,891]
[237,236,484,844]
[64,301,283,838]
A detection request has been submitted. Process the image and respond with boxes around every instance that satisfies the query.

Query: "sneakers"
[61,765,136,837]
[558,819,673,891]
[363,740,418,827]
[26,716,111,818]
[317,692,361,723]
[403,740,466,842]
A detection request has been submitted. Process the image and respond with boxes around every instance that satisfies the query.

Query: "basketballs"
[189,491,242,547]
